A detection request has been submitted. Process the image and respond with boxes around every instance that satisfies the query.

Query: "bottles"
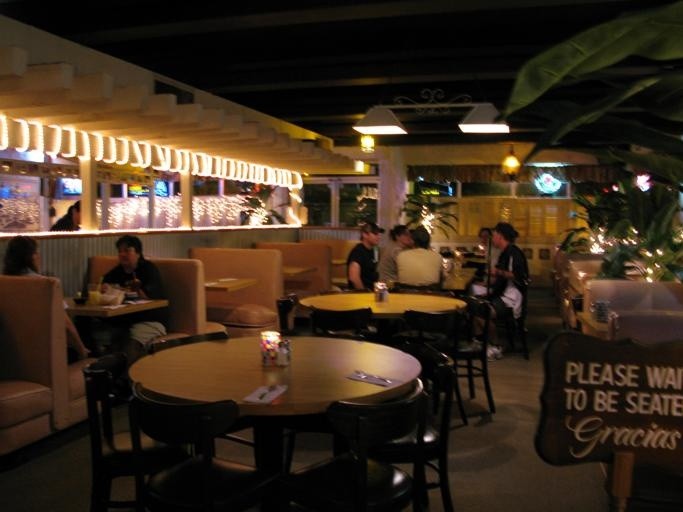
[374,282,388,303]
[128,272,140,294]
[276,339,290,365]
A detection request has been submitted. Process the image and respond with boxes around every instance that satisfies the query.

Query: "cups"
[595,300,608,323]
[68,275,125,305]
[258,332,279,365]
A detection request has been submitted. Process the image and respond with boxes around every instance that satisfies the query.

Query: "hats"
[360,222,385,233]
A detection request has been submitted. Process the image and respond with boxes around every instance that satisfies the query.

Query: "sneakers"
[469,339,503,362]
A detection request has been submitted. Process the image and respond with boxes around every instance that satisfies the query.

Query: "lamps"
[352,101,510,135]
[500,143,522,183]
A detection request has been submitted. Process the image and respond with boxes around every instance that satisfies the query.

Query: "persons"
[90,234,169,401]
[7,236,103,415]
[51,200,82,232]
[347,223,529,361]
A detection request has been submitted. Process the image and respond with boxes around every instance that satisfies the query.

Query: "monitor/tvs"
[60,176,81,199]
[121,181,174,198]
